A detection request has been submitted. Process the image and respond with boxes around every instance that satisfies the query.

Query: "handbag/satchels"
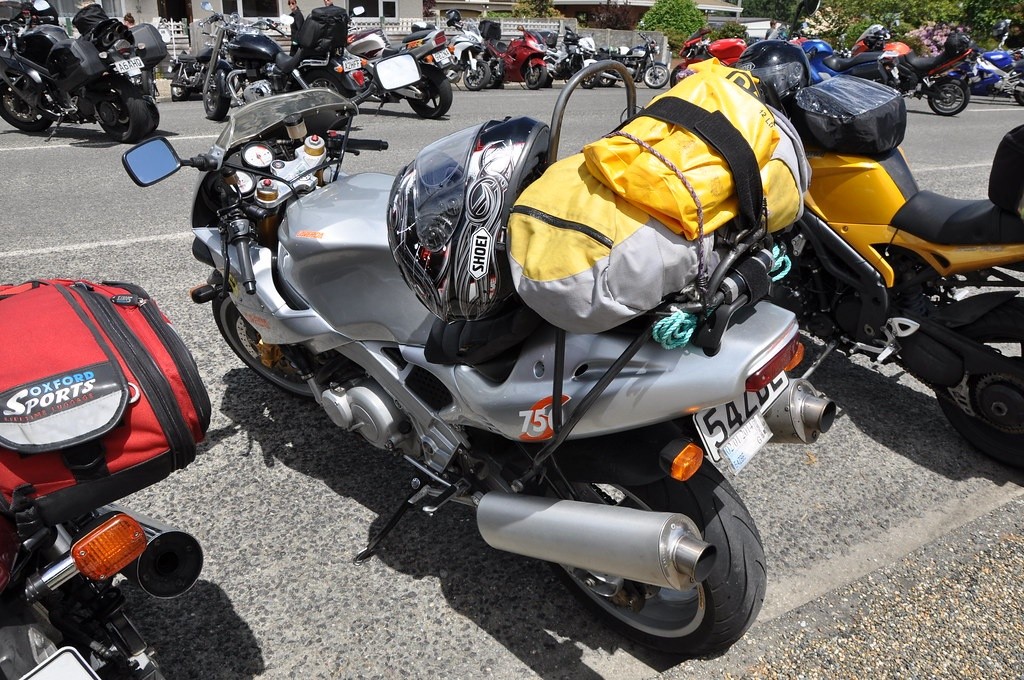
[71,3,109,36]
[503,70,812,335]
[479,16,502,40]
[1,277,212,540]
[297,6,352,54]
[792,71,908,157]
[944,30,971,56]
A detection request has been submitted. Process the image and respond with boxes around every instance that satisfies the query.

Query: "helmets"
[385,115,551,325]
[445,9,461,27]
[991,19,1008,41]
[737,39,812,124]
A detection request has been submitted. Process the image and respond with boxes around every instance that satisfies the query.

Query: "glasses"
[288,2,295,5]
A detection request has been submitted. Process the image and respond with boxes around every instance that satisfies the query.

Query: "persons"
[765,20,777,40]
[0,0,135,29]
[288,0,334,56]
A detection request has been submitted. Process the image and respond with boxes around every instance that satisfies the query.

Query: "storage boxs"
[945,32,970,56]
[19,23,71,65]
[131,22,169,69]
[46,41,106,95]
[298,6,349,55]
[478,20,502,41]
[538,30,557,48]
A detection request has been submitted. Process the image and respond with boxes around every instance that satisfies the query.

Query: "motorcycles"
[156,43,233,102]
[947,42,1024,104]
[120,90,838,658]
[342,28,455,116]
[668,27,752,89]
[792,31,887,99]
[1,0,174,142]
[496,27,553,86]
[600,32,667,85]
[440,19,492,90]
[531,25,619,87]
[670,0,1024,463]
[828,9,972,119]
[198,14,368,122]
[0,482,211,680]
